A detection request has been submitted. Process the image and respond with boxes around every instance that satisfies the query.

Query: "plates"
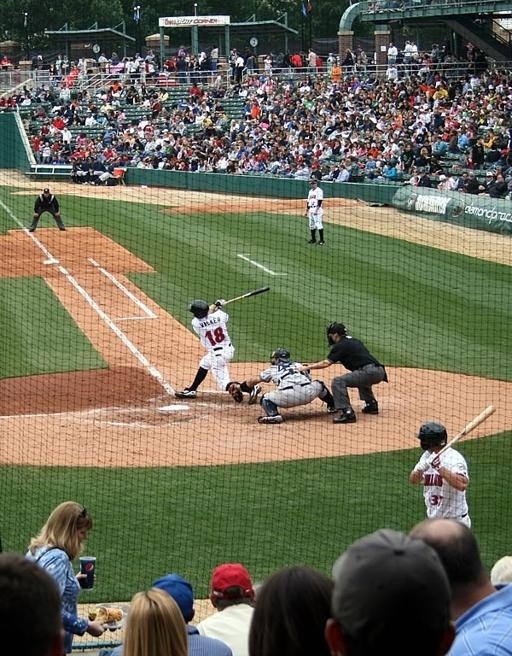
[82,602,131,630]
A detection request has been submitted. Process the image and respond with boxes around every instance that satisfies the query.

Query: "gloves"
[426,454,445,471]
[415,462,432,474]
[214,299,226,309]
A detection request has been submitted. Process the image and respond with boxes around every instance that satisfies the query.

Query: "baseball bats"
[223,286,270,305]
[437,405,496,457]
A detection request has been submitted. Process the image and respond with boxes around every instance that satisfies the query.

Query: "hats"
[151,573,195,621]
[208,561,256,599]
[328,526,450,647]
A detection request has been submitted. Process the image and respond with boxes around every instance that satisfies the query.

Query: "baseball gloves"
[228,383,243,402]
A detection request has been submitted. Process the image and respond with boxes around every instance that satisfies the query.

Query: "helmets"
[412,422,447,449]
[269,348,292,363]
[187,299,209,318]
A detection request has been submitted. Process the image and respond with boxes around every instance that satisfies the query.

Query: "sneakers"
[175,388,198,399]
[257,414,283,425]
[361,401,379,414]
[246,382,262,404]
[331,410,356,424]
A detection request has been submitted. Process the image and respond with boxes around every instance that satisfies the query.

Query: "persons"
[302,180,325,245]
[297,322,386,424]
[29,188,65,231]
[234,348,337,424]
[1,501,511,656]
[175,300,261,404]
[409,424,471,534]
[1,40,510,186]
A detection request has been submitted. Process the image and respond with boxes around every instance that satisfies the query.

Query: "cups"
[78,556,97,591]
